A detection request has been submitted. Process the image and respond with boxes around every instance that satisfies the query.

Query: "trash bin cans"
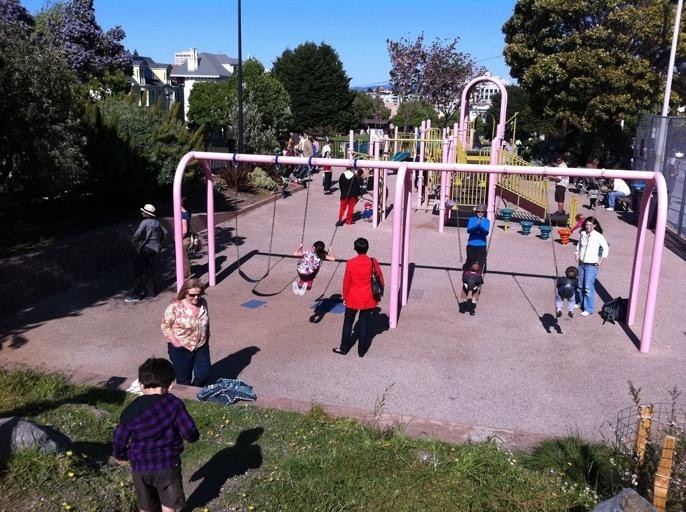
[631,183,669,229]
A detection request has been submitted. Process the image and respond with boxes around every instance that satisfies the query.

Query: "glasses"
[477,211,485,213]
[186,292,203,297]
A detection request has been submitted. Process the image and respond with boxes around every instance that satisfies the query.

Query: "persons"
[569,213,588,232]
[557,264,580,317]
[331,237,385,355]
[110,358,202,512]
[292,240,336,296]
[159,277,211,387]
[130,203,168,300]
[498,137,680,221]
[575,217,609,318]
[462,259,483,305]
[466,204,492,272]
[278,131,389,228]
[180,198,193,280]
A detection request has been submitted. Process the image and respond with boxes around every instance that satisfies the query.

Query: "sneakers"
[568,311,575,317]
[292,281,299,295]
[125,295,140,302]
[557,311,563,317]
[299,282,308,296]
[580,311,589,316]
[333,348,345,355]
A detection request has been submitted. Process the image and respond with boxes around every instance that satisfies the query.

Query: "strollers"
[576,177,606,196]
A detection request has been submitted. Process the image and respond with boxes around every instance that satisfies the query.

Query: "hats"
[365,202,371,206]
[140,204,156,217]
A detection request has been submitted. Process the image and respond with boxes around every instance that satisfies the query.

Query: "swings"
[234,153,280,283]
[297,157,358,281]
[542,175,605,301]
[453,172,507,295]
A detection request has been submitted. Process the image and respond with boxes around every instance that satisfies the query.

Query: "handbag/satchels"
[372,271,383,297]
[182,235,201,255]
[602,297,628,322]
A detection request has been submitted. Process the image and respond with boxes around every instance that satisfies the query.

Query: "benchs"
[618,197,632,212]
